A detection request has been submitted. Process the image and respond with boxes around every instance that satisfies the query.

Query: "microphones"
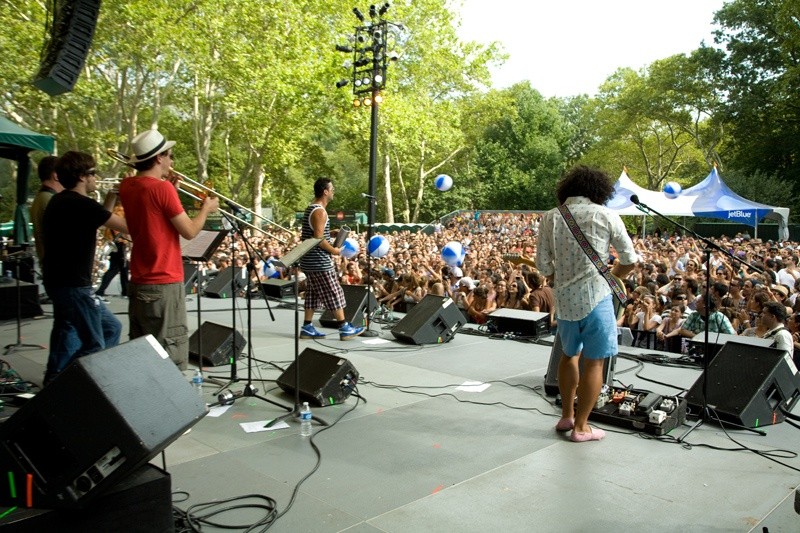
[224,200,239,212]
[360,192,376,199]
[631,195,640,205]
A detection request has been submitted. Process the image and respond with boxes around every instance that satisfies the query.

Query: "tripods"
[3,245,46,356]
[207,208,330,428]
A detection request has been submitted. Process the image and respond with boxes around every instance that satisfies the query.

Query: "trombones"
[95,177,127,193]
[167,165,296,247]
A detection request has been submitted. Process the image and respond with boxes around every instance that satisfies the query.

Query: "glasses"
[87,170,95,176]
[160,154,173,160]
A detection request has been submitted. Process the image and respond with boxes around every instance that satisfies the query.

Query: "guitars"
[499,252,630,306]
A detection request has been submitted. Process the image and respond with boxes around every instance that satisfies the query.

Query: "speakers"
[32,0,102,96]
[320,283,378,330]
[259,279,296,298]
[689,331,775,363]
[389,294,467,347]
[684,340,800,428]
[0,333,210,511]
[190,322,247,368]
[204,266,249,298]
[275,347,359,406]
[182,264,204,295]
[487,308,550,336]
[543,330,620,393]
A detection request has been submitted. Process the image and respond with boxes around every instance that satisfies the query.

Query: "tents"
[601,165,699,240]
[679,161,789,242]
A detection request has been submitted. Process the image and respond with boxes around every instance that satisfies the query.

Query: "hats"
[128,130,176,163]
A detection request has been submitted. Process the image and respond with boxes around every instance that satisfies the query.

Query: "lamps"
[352,7,364,21]
[369,5,375,18]
[353,93,362,107]
[336,79,349,88]
[336,45,352,53]
[379,3,390,15]
[363,77,370,85]
[385,51,399,66]
[354,79,362,87]
[353,27,387,66]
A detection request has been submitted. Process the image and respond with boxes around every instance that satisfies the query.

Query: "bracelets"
[742,319,749,323]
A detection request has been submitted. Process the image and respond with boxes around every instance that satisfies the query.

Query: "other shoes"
[571,425,605,441]
[555,419,574,430]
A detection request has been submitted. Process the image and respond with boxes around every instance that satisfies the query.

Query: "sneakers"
[338,322,365,340]
[300,324,326,339]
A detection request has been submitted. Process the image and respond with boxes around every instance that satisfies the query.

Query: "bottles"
[300,402,312,437]
[192,369,202,395]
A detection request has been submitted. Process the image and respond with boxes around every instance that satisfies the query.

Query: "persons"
[41,151,130,372]
[535,164,639,442]
[117,129,220,371]
[300,177,365,341]
[677,294,738,339]
[95,183,130,299]
[91,209,800,352]
[31,155,82,386]
[760,301,794,359]
